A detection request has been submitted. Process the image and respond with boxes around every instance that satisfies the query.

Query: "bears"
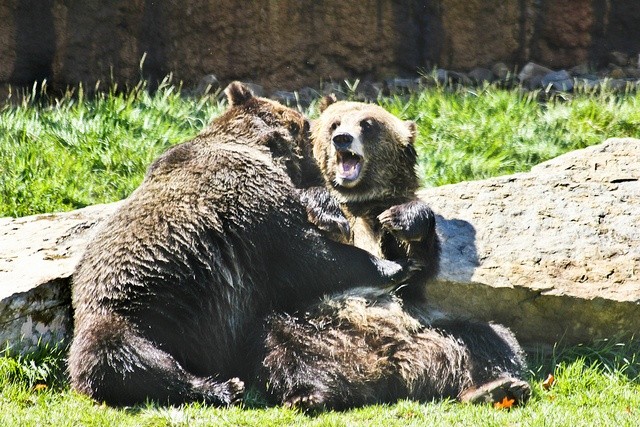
[240,92,534,416]
[66,80,427,410]
